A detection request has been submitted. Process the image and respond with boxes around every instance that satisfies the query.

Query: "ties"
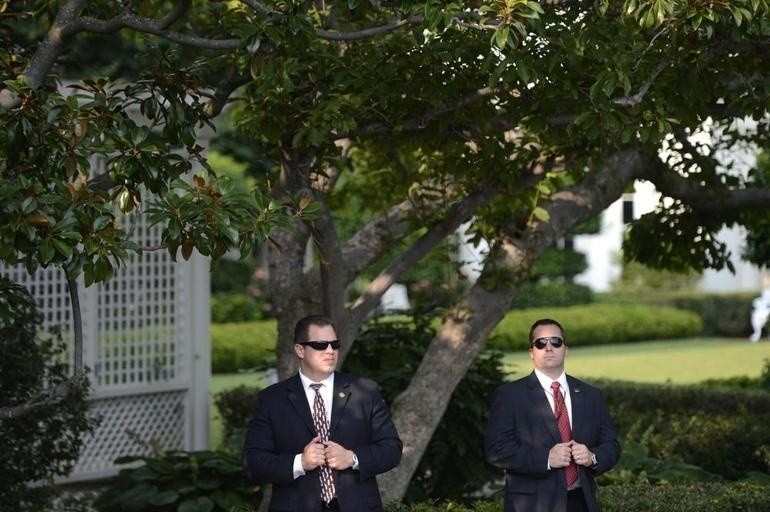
[551,381,579,487]
[309,383,337,509]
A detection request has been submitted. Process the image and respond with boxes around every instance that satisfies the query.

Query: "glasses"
[533,337,564,349]
[300,340,342,350]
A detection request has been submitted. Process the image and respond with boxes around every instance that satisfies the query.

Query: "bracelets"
[351,451,357,464]
[590,452,597,466]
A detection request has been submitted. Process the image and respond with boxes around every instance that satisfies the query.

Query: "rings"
[577,455,580,459]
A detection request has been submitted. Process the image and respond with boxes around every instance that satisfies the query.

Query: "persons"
[486,317,622,511]
[243,315,404,511]
